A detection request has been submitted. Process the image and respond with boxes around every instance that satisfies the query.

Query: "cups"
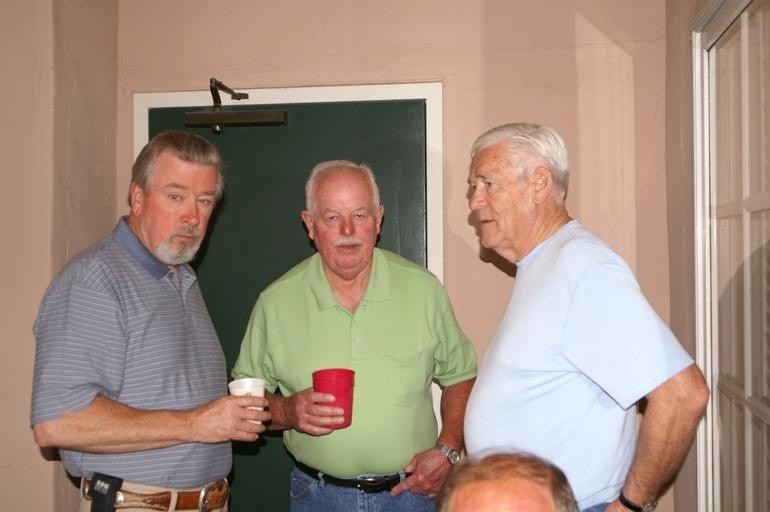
[311,367,356,431]
[227,377,268,430]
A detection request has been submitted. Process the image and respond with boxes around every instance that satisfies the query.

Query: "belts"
[291,449,412,494]
[80,478,230,511]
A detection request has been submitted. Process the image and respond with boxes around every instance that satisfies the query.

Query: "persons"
[463,123,711,512]
[230,161,477,512]
[29,129,272,512]
[433,453,579,512]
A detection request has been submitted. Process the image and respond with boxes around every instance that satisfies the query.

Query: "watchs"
[617,493,657,511]
[434,442,461,466]
